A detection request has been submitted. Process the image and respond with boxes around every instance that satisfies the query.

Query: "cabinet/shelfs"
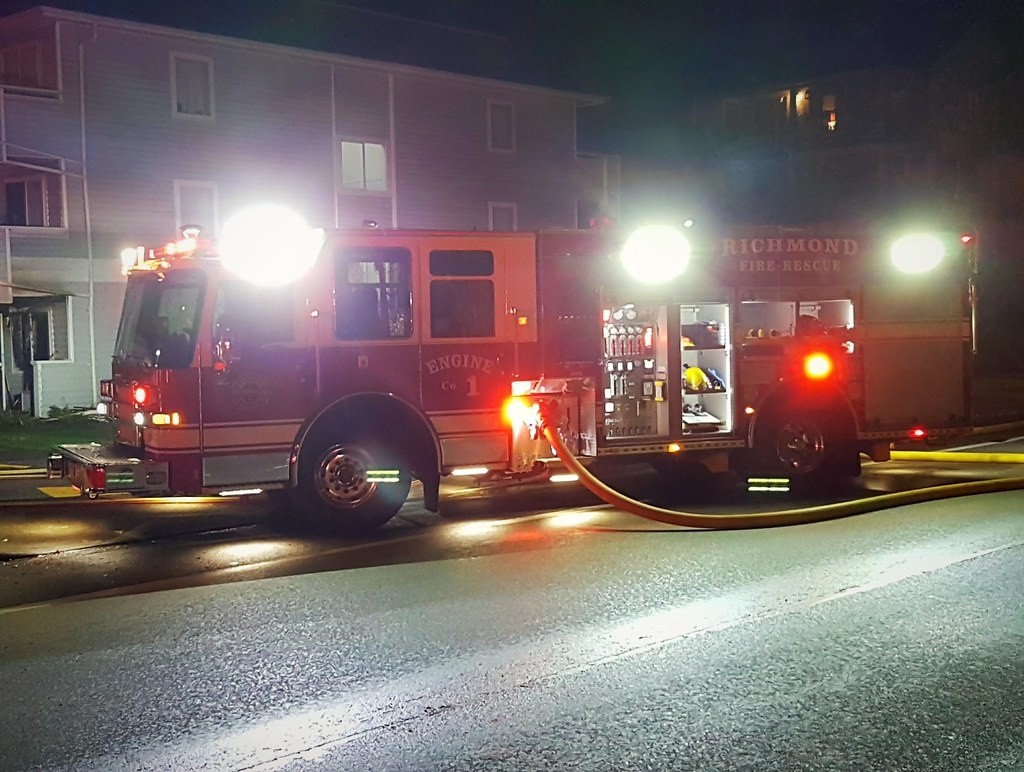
[675,306,733,435]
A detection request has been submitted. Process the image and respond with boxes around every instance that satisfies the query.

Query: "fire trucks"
[47,214,1024,534]
